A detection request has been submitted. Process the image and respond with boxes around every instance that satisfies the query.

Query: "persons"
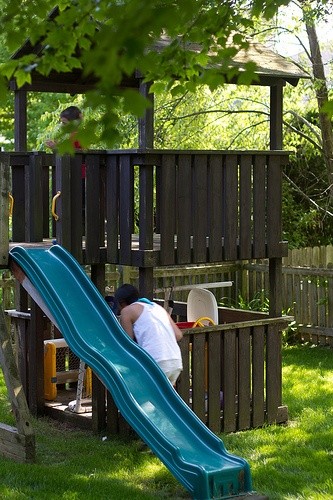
[113,283,184,388]
[45,105,87,245]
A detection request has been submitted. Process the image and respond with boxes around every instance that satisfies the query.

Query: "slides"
[9,244,253,500]
